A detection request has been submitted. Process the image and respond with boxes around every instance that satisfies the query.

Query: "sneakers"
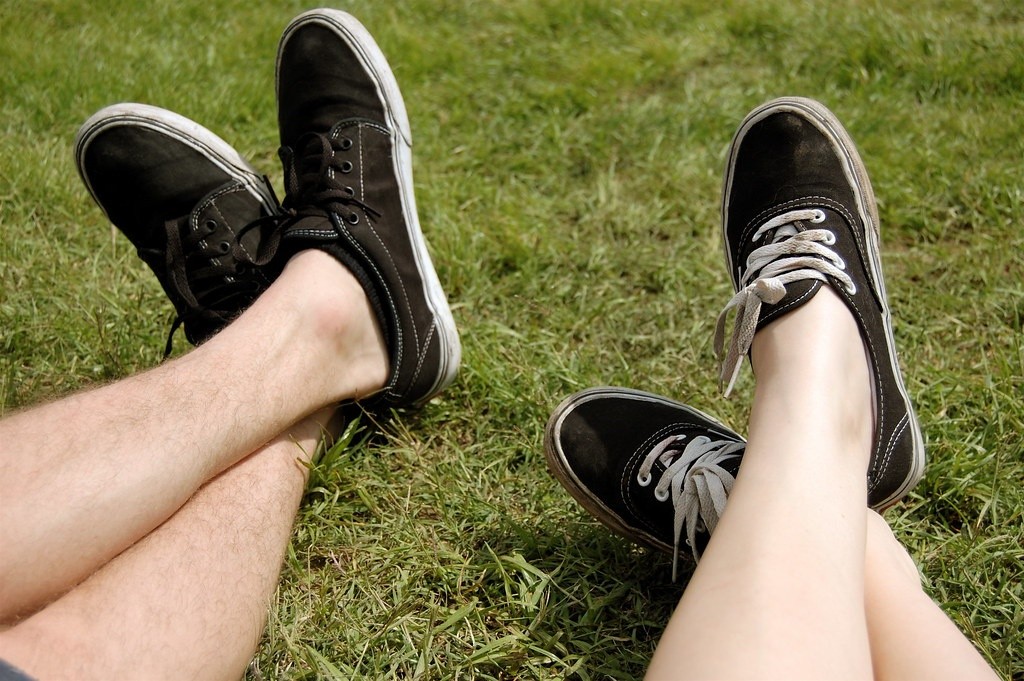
[73,103,390,450]
[545,386,749,585]
[231,8,462,413]
[713,100,925,515]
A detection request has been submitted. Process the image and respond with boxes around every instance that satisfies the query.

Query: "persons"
[0,8,462,681]
[543,96,1002,681]
